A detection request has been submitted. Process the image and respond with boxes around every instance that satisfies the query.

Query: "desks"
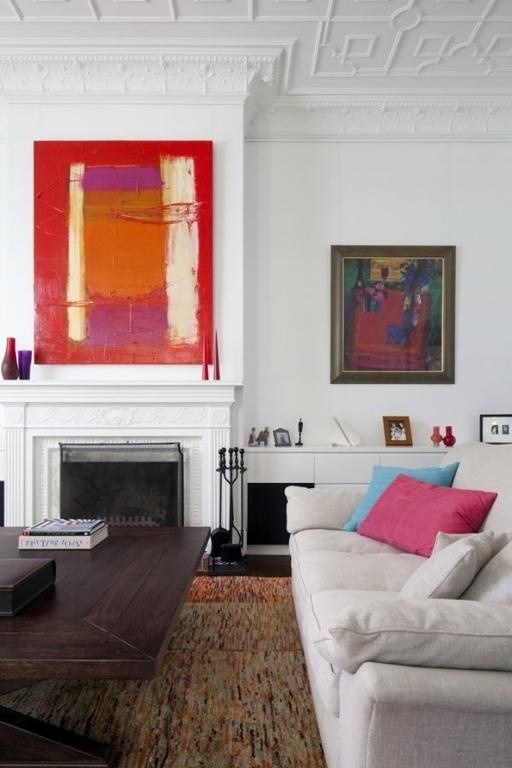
[0,520,214,767]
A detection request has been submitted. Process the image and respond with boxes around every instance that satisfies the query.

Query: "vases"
[444,425,457,447]
[16,349,34,382]
[431,426,444,447]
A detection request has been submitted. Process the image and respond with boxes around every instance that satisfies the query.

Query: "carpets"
[236,553,293,579]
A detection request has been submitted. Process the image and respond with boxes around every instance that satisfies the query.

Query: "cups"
[18,351,32,380]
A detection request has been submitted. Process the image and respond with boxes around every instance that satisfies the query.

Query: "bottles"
[443,425,456,447]
[431,426,443,445]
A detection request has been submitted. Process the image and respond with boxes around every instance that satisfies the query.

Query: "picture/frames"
[327,242,458,388]
[382,414,414,447]
[478,412,512,446]
[273,428,292,447]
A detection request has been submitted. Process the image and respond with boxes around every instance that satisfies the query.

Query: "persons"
[390,422,406,440]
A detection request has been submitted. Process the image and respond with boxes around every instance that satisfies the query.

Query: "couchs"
[280,438,512,766]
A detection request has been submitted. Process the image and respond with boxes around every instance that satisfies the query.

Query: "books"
[17,518,110,550]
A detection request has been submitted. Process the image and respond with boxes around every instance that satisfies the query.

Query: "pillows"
[355,474,498,562]
[342,462,464,533]
[397,529,510,609]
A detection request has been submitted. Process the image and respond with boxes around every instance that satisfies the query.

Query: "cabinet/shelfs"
[310,448,380,493]
[376,446,458,475]
[238,447,316,558]
